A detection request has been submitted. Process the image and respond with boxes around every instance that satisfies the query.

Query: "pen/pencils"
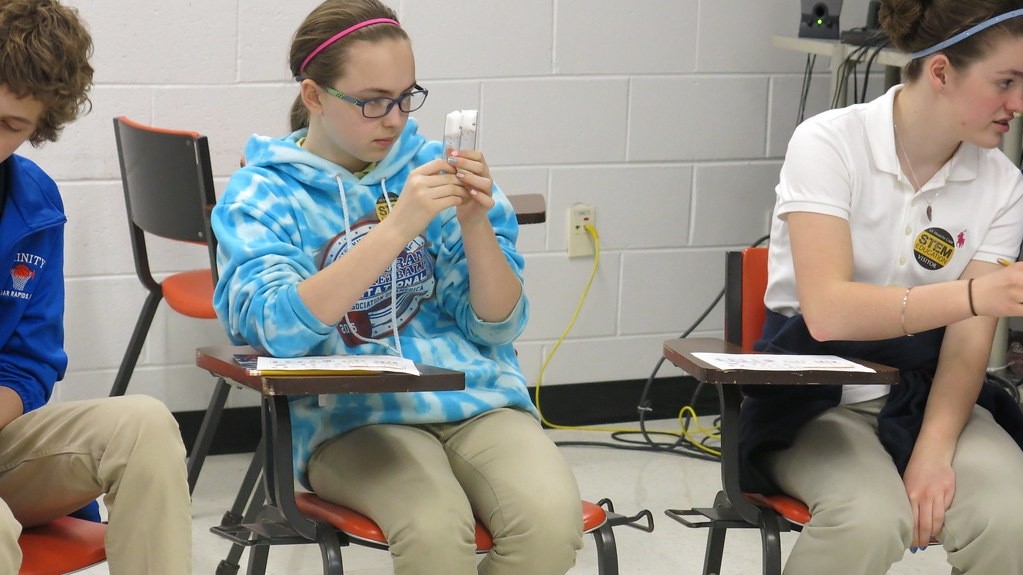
[997,257,1012,267]
[245,367,385,378]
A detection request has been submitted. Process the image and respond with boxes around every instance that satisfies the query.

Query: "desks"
[663,337,906,531]
[192,333,469,540]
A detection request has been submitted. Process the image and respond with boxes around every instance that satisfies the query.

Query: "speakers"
[798,0,843,39]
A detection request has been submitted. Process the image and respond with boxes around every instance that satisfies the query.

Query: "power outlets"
[566,203,598,258]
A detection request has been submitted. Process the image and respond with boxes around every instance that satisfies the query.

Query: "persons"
[0,0,193,574]
[737,0,1023,575]
[210,1,585,575]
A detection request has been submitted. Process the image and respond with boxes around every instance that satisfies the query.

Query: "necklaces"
[893,120,956,221]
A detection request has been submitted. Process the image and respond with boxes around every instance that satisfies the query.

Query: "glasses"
[320,83,429,119]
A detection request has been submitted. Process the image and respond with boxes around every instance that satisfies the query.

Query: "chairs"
[18,515,108,575]
[700,240,1020,575]
[110,111,241,494]
[249,438,634,575]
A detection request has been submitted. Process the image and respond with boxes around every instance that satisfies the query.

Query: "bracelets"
[901,286,916,338]
[968,277,979,316]
